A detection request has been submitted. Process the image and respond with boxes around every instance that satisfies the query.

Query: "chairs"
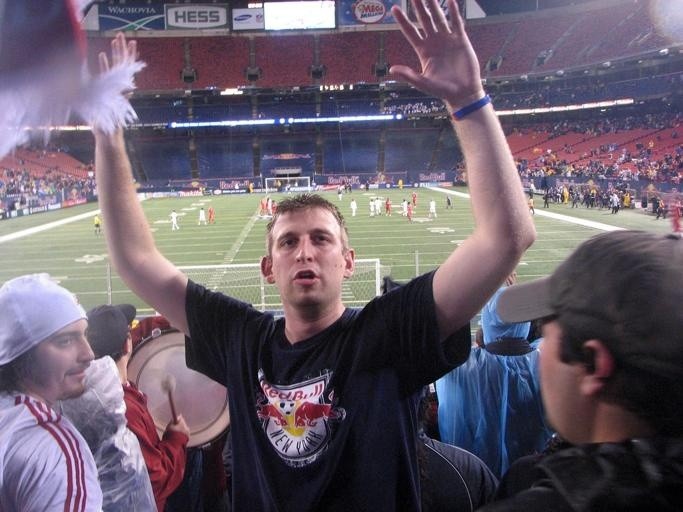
[85,0,681,93]
[0,149,94,183]
[505,122,682,182]
[128,95,468,184]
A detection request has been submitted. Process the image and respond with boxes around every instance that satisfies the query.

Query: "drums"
[127,328,230,449]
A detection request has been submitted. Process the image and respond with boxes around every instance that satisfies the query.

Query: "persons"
[0,0,683,512]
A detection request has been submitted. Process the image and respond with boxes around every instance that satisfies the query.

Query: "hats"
[0,273,90,366]
[84,303,136,352]
[496,230,683,375]
